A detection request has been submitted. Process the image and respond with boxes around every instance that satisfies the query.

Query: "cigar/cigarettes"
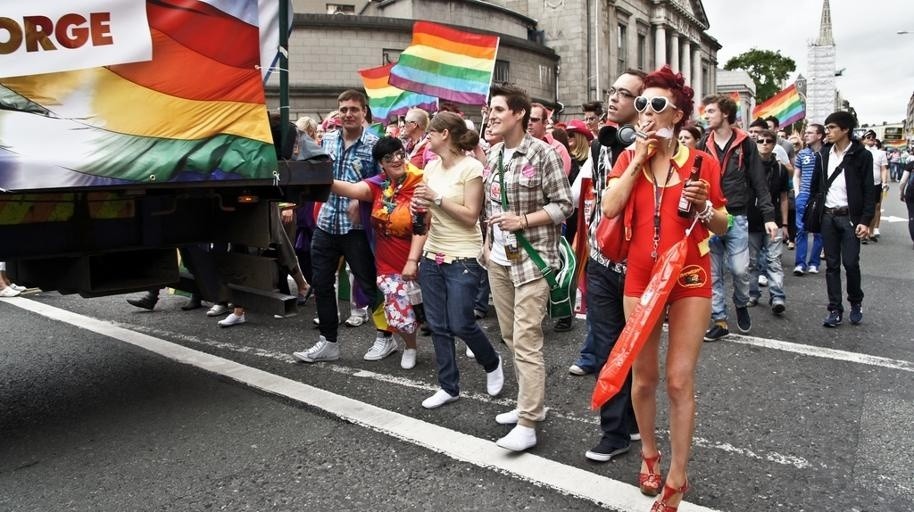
[482,219,494,224]
[768,236,782,240]
[642,122,650,127]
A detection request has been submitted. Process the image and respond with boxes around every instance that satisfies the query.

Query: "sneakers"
[495,425,537,452]
[861,237,869,244]
[297,285,313,306]
[292,336,340,362]
[206,303,229,316]
[552,316,575,332]
[312,312,342,326]
[822,309,843,327]
[787,242,795,251]
[758,273,768,286]
[584,437,632,462]
[421,388,460,410]
[703,325,730,343]
[793,264,806,274]
[495,408,547,424]
[217,311,246,326]
[849,300,864,324]
[363,332,398,361]
[746,296,758,307]
[734,305,753,333]
[874,228,880,237]
[486,354,505,396]
[770,297,787,314]
[808,265,819,273]
[400,347,417,369]
[180,295,202,310]
[568,363,588,376]
[126,294,159,310]
[344,305,370,327]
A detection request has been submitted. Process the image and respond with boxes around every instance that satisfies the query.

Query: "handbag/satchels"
[498,143,577,320]
[594,149,636,263]
[800,142,858,234]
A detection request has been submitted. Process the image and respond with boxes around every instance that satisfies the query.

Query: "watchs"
[434,194,442,209]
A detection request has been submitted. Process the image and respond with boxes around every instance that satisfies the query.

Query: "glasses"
[633,95,676,114]
[568,132,575,138]
[606,88,635,98]
[756,139,773,144]
[866,136,876,139]
[427,129,439,134]
[403,119,415,124]
[383,152,406,161]
[530,117,541,123]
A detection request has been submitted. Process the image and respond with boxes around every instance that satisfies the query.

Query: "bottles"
[412,204,429,237]
[501,229,521,261]
[678,154,705,218]
[408,279,429,326]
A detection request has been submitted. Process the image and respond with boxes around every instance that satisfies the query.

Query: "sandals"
[638,449,663,496]
[649,473,689,511]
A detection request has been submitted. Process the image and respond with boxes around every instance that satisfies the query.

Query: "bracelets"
[695,200,715,223]
[522,212,529,227]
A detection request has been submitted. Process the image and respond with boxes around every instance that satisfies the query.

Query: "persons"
[296,117,317,139]
[792,124,825,274]
[748,117,793,175]
[128,289,201,310]
[270,201,312,306]
[898,159,913,249]
[873,138,883,236]
[569,154,599,376]
[479,106,490,140]
[322,111,342,134]
[313,254,369,326]
[399,116,412,149]
[484,84,575,459]
[764,115,793,174]
[788,135,803,249]
[678,126,701,150]
[207,302,246,327]
[474,118,501,319]
[602,68,727,512]
[385,124,397,139]
[294,88,399,364]
[695,94,778,341]
[777,130,787,139]
[423,104,487,168]
[694,124,705,134]
[404,109,429,169]
[747,129,788,311]
[525,103,571,177]
[862,130,888,244]
[586,68,647,461]
[409,111,504,409]
[810,111,874,327]
[330,137,430,370]
[582,101,602,140]
[888,148,898,181]
[554,118,594,332]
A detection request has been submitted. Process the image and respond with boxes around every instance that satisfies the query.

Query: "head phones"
[597,124,636,150]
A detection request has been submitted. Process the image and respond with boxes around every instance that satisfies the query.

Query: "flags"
[753,84,804,129]
[387,21,497,106]
[356,65,436,122]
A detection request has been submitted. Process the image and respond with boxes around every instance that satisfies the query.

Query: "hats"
[565,119,595,141]
